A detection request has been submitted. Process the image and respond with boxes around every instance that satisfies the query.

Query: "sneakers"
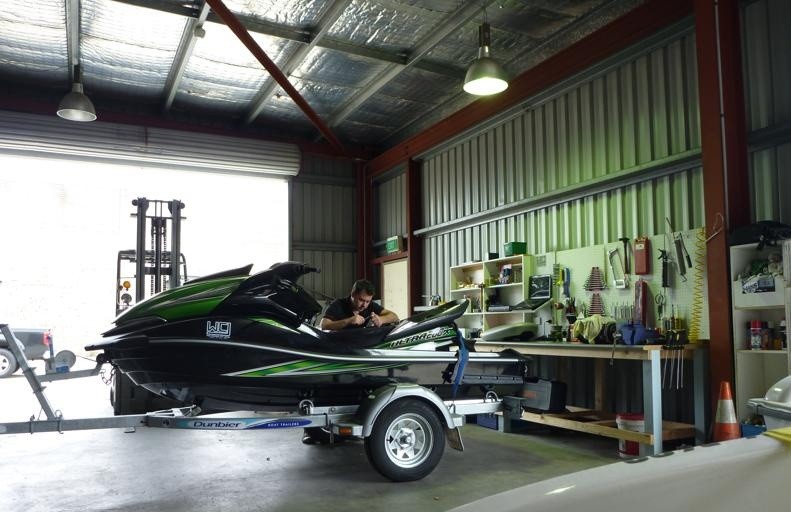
[302,429,339,444]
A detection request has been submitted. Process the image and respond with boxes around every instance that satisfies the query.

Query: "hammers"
[619,238,630,274]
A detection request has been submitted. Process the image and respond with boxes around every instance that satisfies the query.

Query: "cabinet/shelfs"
[449,253,535,337]
[729,240,791,429]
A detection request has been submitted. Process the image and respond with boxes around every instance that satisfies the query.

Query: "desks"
[471,337,710,460]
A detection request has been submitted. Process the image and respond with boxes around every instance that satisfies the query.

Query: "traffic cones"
[712,381,741,443]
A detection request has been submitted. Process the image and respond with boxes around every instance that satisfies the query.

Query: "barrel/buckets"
[616,413,647,459]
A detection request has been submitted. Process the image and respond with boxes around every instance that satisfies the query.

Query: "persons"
[302,278,399,444]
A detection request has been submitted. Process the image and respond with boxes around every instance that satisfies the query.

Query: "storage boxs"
[503,241,527,256]
[524,376,568,413]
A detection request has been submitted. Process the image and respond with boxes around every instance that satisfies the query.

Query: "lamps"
[460,0,510,98]
[54,63,98,123]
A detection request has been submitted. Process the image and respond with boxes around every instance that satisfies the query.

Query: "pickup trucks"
[0,328,51,378]
